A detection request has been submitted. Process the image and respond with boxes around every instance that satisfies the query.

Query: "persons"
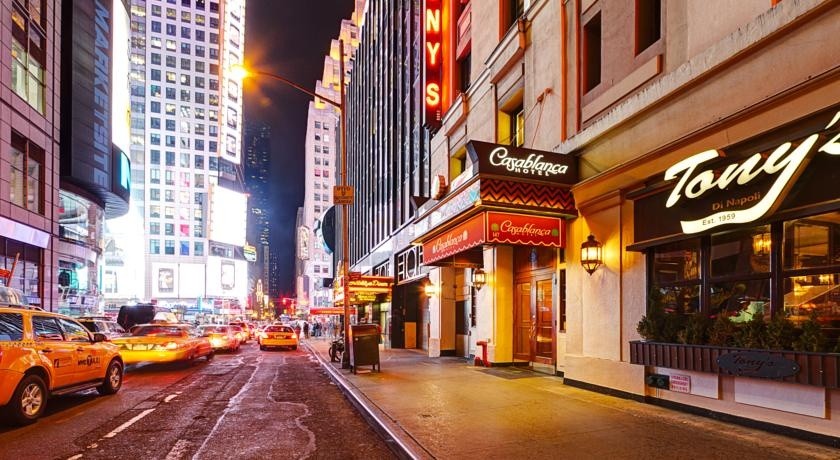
[186,314,342,341]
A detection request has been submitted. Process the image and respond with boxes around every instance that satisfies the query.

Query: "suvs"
[0,306,126,421]
[76,315,123,341]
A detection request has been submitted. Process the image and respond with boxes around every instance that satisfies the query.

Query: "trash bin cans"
[349,325,380,375]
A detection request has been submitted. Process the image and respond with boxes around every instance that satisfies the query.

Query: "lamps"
[472,265,487,292]
[581,233,603,275]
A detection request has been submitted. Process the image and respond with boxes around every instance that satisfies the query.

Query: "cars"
[196,320,316,353]
[113,322,213,367]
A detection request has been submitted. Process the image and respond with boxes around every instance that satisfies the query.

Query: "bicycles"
[327,341,343,361]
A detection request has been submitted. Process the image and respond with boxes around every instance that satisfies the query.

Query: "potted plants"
[629,309,840,389]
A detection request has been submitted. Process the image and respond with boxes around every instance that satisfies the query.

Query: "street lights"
[231,38,354,368]
